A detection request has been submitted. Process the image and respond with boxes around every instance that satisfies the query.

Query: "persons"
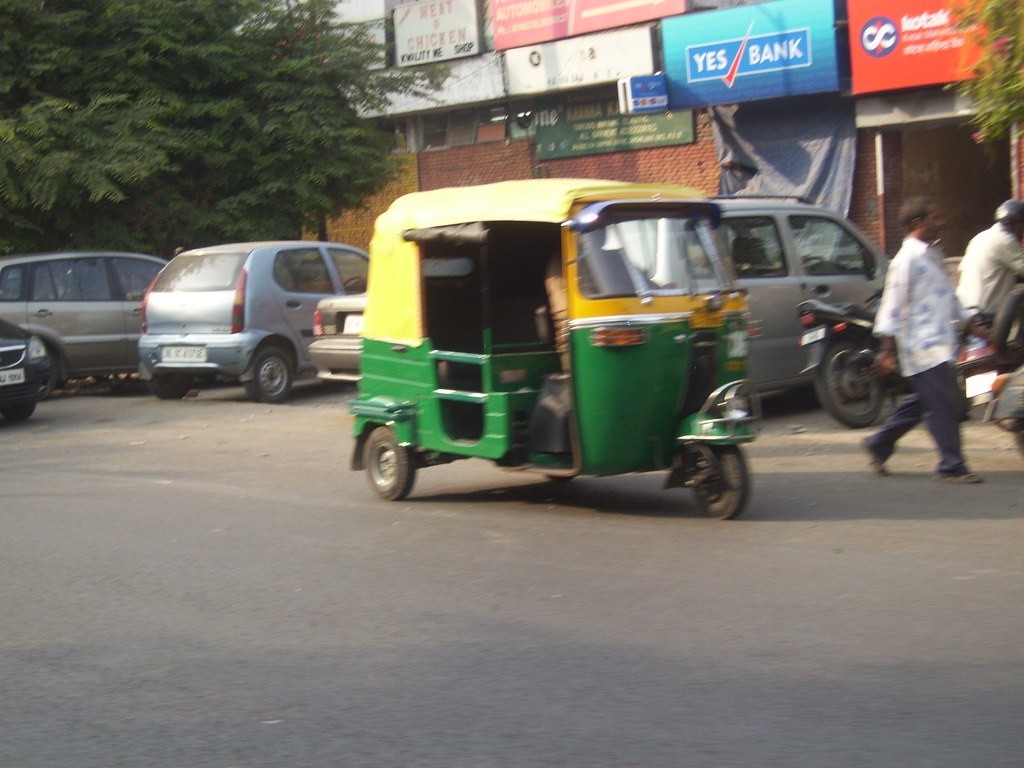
[954,199,1024,342]
[864,196,984,484]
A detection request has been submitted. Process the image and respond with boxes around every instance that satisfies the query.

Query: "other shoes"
[932,473,984,483]
[861,442,891,477]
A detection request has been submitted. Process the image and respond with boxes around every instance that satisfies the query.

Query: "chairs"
[298,258,326,292]
[733,236,777,274]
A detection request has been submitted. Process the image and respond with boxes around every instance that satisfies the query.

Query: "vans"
[0,250,169,378]
[706,197,928,417]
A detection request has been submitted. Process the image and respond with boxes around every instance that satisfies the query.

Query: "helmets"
[995,199,1024,225]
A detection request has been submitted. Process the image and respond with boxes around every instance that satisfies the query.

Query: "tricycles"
[343,178,754,521]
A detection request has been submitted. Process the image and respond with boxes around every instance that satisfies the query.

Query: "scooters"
[795,283,1024,430]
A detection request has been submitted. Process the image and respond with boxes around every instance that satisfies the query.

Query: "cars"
[309,291,366,388]
[0,319,49,419]
[138,239,370,402]
